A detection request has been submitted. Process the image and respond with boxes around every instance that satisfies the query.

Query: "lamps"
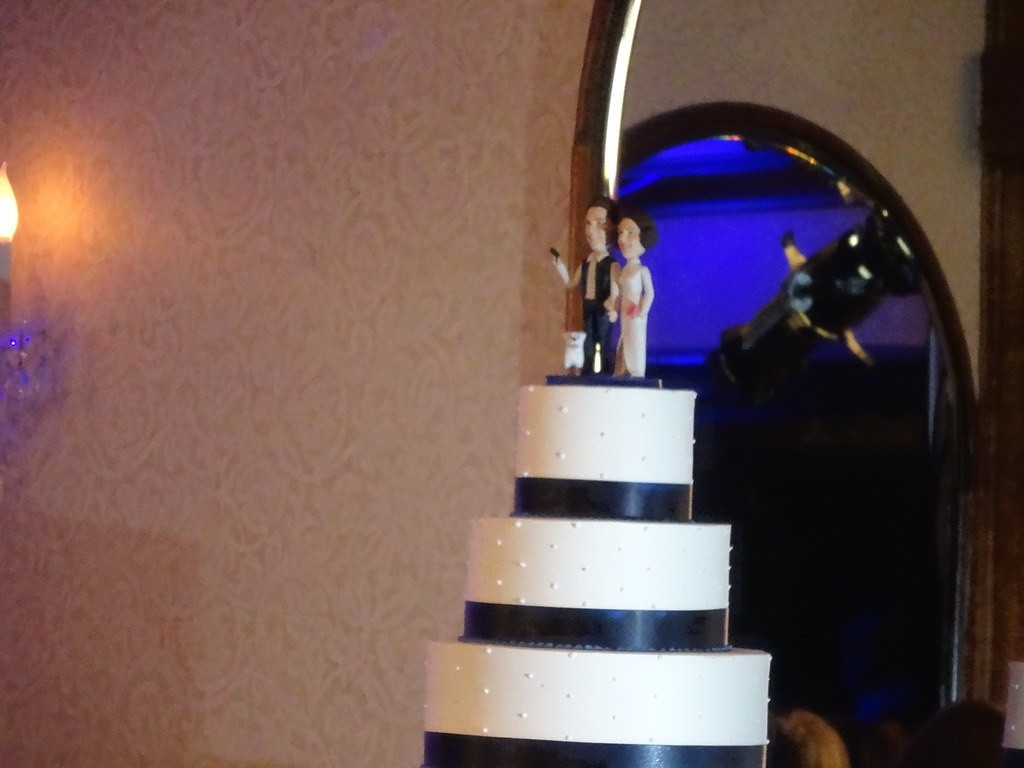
[0,161,58,462]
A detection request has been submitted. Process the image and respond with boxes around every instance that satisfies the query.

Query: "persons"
[767,697,1008,768]
[546,195,621,377]
[604,206,659,380]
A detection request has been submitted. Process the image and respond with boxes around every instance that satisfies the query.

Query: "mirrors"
[619,102,980,768]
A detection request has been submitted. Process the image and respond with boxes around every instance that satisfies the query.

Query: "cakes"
[419,197,774,768]
